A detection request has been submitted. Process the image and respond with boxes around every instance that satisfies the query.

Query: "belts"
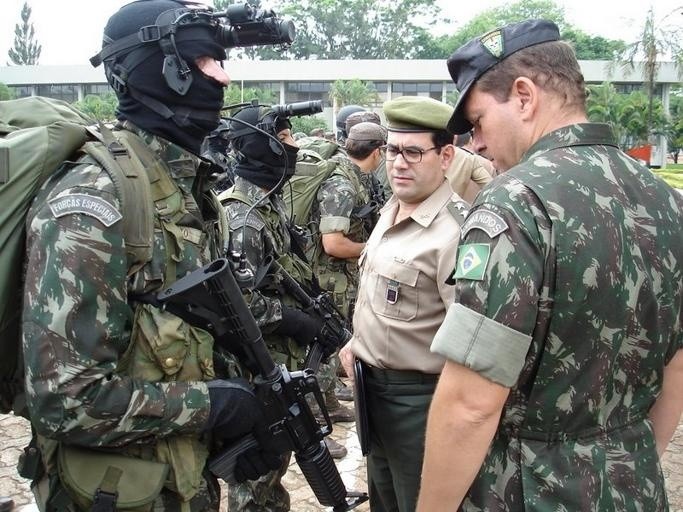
[362,361,436,385]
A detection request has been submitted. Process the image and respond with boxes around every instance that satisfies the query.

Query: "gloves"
[288,311,317,347]
[321,334,338,365]
[235,425,283,482]
[205,378,263,439]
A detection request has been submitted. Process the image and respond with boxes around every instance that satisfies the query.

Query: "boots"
[320,390,354,423]
[334,376,354,401]
[315,412,346,459]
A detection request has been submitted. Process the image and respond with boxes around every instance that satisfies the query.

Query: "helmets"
[336,105,365,132]
[205,118,230,139]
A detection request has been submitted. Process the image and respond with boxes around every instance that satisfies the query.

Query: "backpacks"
[294,136,350,157]
[282,150,372,239]
[0,97,152,413]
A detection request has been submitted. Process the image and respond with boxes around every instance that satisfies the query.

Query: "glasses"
[379,143,436,165]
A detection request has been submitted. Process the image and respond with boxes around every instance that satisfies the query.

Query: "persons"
[336,96,475,512]
[219,100,340,512]
[197,99,496,202]
[289,124,391,459]
[414,12,683,511]
[18,1,296,512]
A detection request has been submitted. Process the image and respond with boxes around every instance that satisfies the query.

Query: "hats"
[346,112,381,131]
[349,122,386,143]
[382,95,454,134]
[445,17,560,136]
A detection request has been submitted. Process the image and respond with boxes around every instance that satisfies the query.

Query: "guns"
[159,256,369,512]
[356,199,383,233]
[251,252,354,381]
